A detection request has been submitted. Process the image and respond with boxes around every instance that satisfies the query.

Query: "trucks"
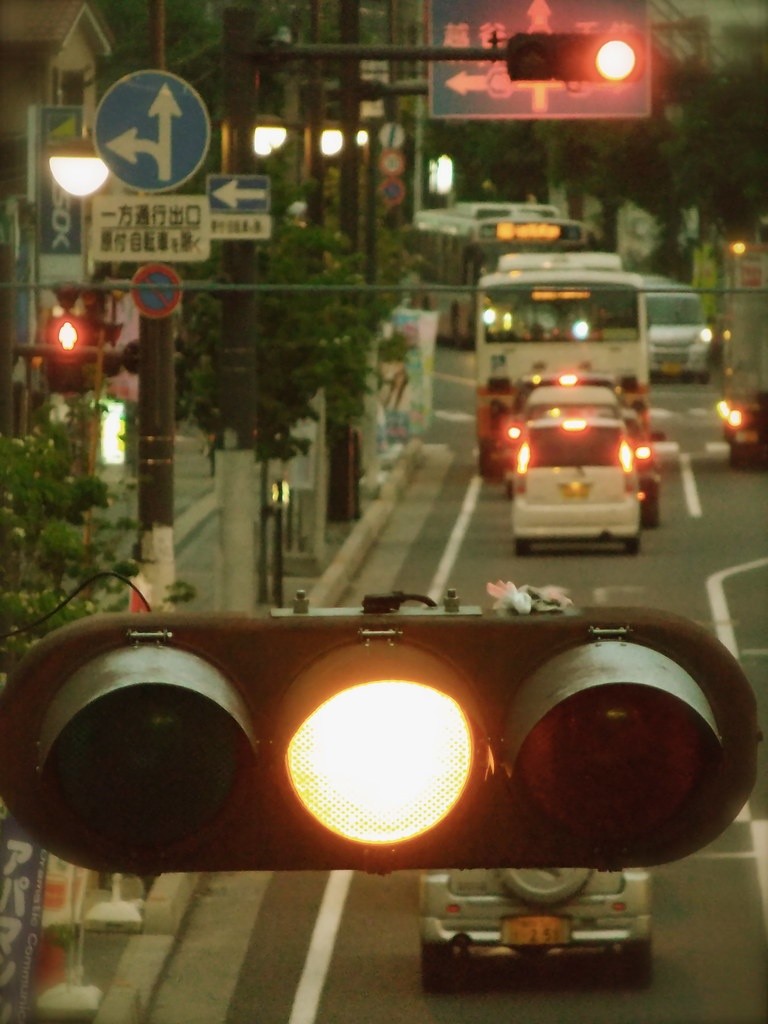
[717,288,768,469]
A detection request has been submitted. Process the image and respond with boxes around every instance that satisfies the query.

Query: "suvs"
[416,866,651,992]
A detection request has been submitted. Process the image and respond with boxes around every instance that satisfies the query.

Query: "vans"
[644,279,710,382]
[509,418,641,557]
[514,376,661,533]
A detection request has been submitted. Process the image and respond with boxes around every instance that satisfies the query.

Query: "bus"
[403,199,588,350]
[476,252,651,486]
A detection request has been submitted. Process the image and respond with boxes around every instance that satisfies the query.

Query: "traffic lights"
[505,32,647,84]
[0,609,758,872]
[50,318,88,373]
